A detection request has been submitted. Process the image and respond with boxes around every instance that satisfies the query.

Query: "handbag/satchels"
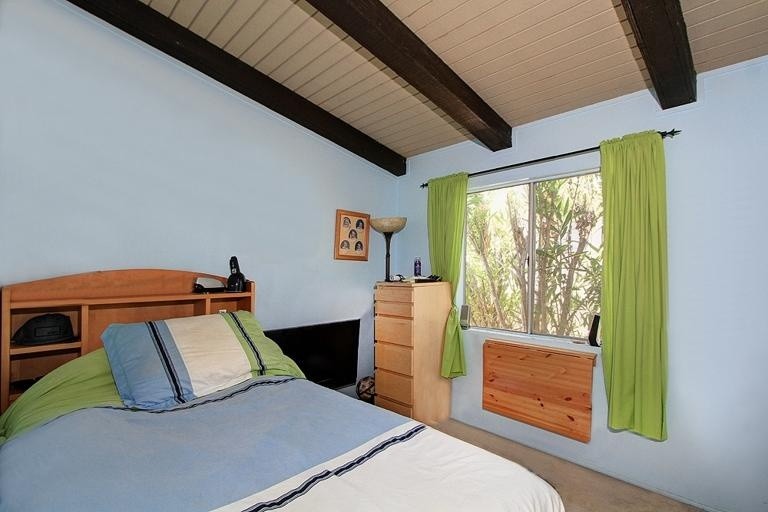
[10,312,76,346]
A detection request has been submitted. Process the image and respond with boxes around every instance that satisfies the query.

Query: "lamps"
[368,216,407,282]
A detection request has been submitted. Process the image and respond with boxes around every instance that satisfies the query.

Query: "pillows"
[99,309,299,412]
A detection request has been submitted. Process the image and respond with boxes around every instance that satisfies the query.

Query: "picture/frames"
[333,208,371,262]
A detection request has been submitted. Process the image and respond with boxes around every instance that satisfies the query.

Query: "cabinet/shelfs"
[374,280,453,425]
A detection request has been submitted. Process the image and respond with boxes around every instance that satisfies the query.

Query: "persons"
[340,216,365,253]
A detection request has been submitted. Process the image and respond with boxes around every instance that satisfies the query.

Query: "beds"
[3,269,563,510]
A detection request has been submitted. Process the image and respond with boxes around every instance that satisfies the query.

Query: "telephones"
[226,256,246,292]
